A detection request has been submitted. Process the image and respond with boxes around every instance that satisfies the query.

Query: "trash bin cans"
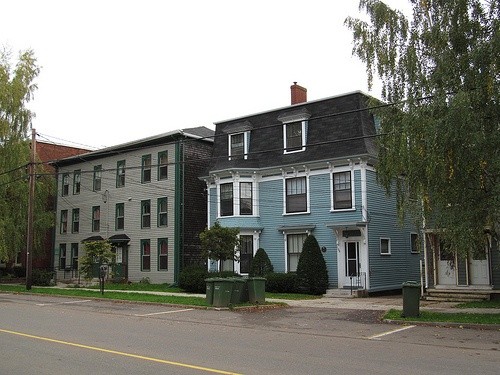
[400,281,421,318]
[204,276,268,308]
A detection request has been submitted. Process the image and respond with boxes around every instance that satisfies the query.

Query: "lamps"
[102,190,110,204]
